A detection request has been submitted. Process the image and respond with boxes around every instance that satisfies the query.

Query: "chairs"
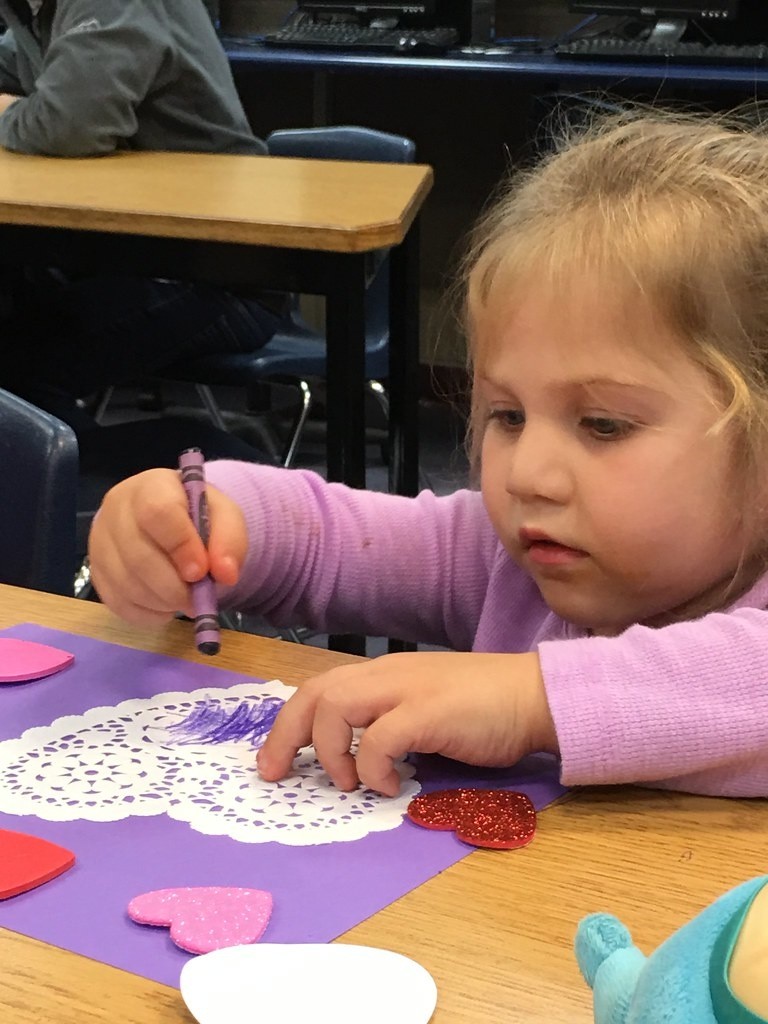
[2,125,417,597]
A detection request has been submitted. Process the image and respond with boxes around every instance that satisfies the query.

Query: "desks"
[0,583,768,1024]
[2,138,435,647]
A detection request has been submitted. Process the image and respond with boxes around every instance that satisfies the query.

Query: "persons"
[0,0,293,482]
[88,88,768,800]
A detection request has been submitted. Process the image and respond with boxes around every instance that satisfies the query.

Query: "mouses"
[397,34,447,58]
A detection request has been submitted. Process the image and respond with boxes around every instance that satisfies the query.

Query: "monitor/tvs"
[297,0,473,35]
[567,0,751,45]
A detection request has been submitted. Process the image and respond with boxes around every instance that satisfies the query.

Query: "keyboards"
[256,22,460,54]
[555,39,768,67]
